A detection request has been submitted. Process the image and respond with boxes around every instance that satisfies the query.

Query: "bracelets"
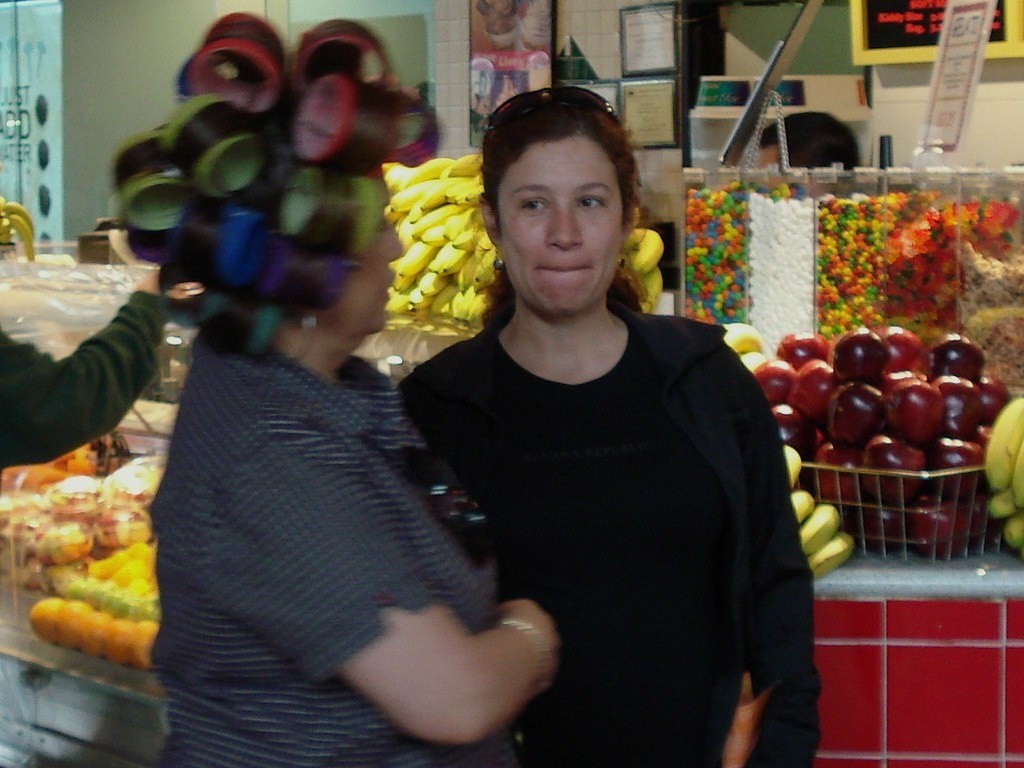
[491,612,556,688]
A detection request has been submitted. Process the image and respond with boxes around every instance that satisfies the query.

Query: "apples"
[756,324,1011,559]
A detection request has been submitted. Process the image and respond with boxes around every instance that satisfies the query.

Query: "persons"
[753,111,862,199]
[400,84,823,768]
[1,270,186,472]
[106,14,559,768]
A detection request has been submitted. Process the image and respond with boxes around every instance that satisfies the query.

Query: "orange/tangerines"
[0,425,165,670]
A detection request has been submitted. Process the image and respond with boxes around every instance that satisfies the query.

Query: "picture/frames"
[466,0,679,149]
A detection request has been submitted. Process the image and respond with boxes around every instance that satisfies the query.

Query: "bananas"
[720,321,856,576]
[986,398,1024,564]
[382,152,665,323]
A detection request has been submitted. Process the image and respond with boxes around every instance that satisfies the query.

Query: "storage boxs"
[75,218,134,265]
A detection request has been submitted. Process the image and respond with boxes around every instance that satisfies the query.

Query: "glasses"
[483,86,620,146]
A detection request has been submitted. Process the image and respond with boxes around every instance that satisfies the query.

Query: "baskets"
[793,462,1004,565]
